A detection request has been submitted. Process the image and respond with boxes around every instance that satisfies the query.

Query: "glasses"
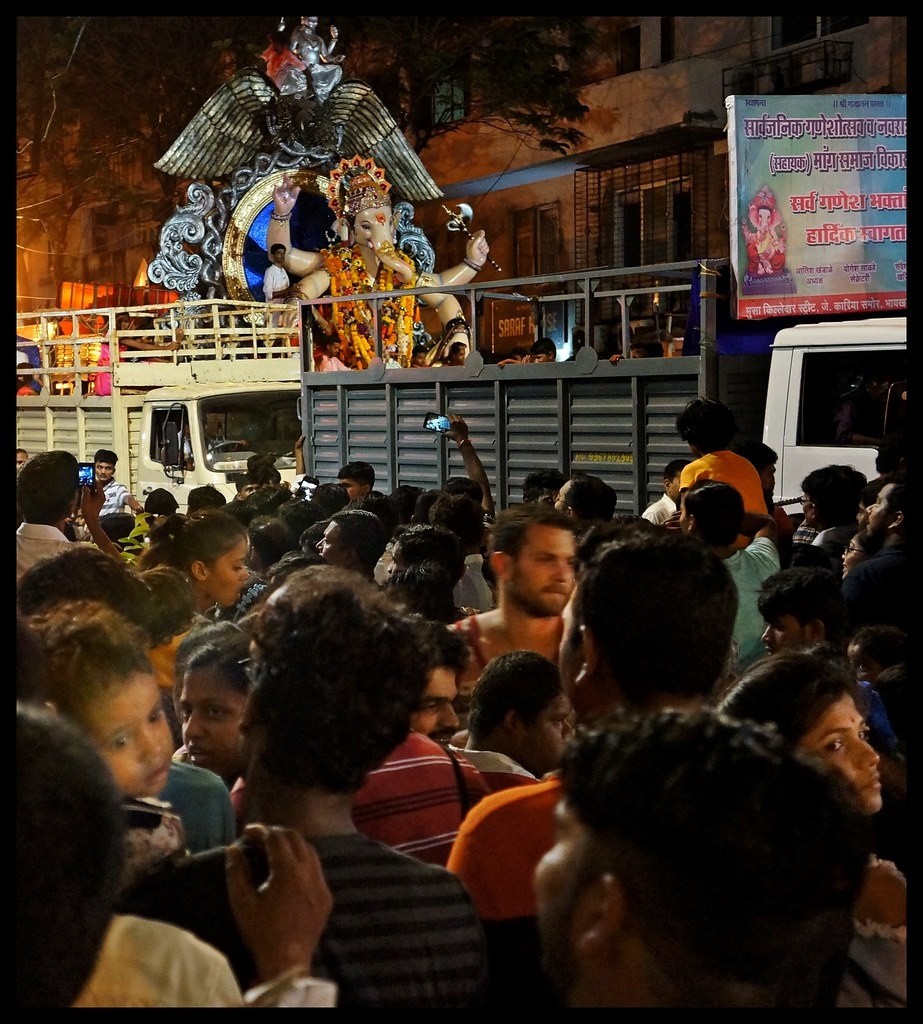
[553,494,568,506]
[797,496,813,505]
[844,546,864,554]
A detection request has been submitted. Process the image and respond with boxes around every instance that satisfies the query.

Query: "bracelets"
[458,440,471,449]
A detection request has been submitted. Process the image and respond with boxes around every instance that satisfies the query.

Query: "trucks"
[298,257,907,519]
[17,298,314,516]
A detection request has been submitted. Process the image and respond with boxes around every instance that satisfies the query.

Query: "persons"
[263,244,673,372]
[447,531,739,1008]
[95,306,180,397]
[15,702,338,1007]
[16,395,910,792]
[277,16,346,103]
[15,600,237,857]
[716,653,907,1007]
[116,566,482,1007]
[464,650,573,791]
[411,618,471,742]
[125,324,169,362]
[16,363,44,395]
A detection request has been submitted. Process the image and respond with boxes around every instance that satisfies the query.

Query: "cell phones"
[423,412,451,434]
[78,463,96,496]
